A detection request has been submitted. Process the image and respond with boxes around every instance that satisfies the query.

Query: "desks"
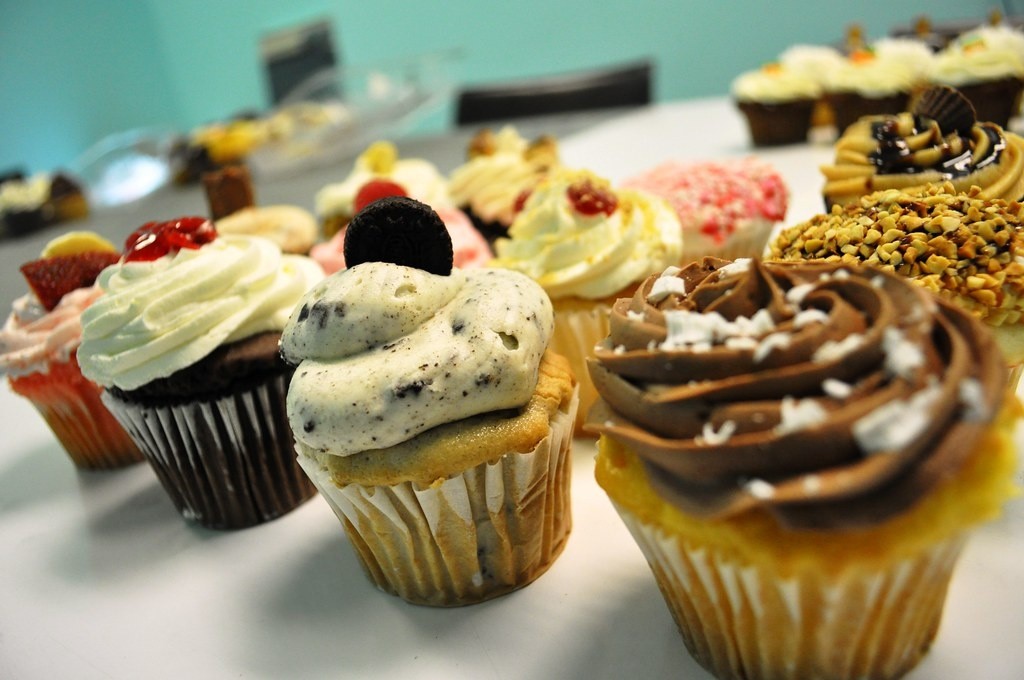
[0,91,1024,680]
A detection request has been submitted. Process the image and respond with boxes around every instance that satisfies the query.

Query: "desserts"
[0,12,1024,679]
